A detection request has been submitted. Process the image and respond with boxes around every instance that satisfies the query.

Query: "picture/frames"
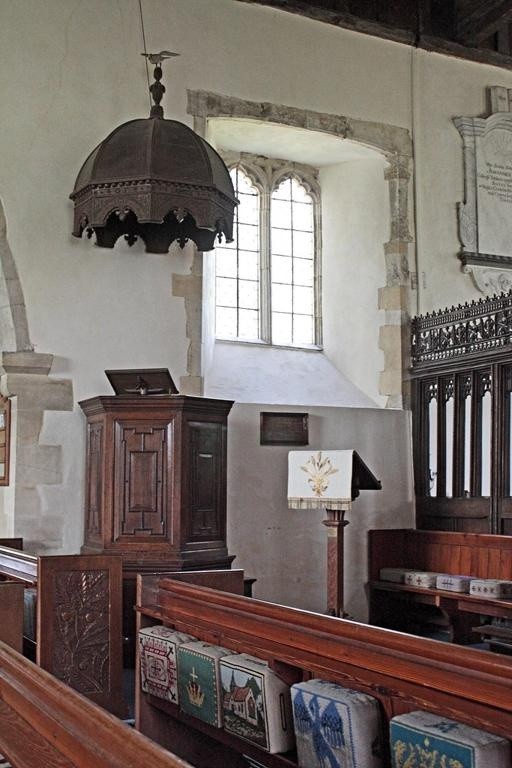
[261,411,310,448]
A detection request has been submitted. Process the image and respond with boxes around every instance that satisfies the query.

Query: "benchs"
[363,529,512,654]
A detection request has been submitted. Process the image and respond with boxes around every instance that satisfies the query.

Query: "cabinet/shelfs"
[81,396,237,666]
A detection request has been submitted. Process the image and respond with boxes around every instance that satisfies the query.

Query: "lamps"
[68,52,236,254]
